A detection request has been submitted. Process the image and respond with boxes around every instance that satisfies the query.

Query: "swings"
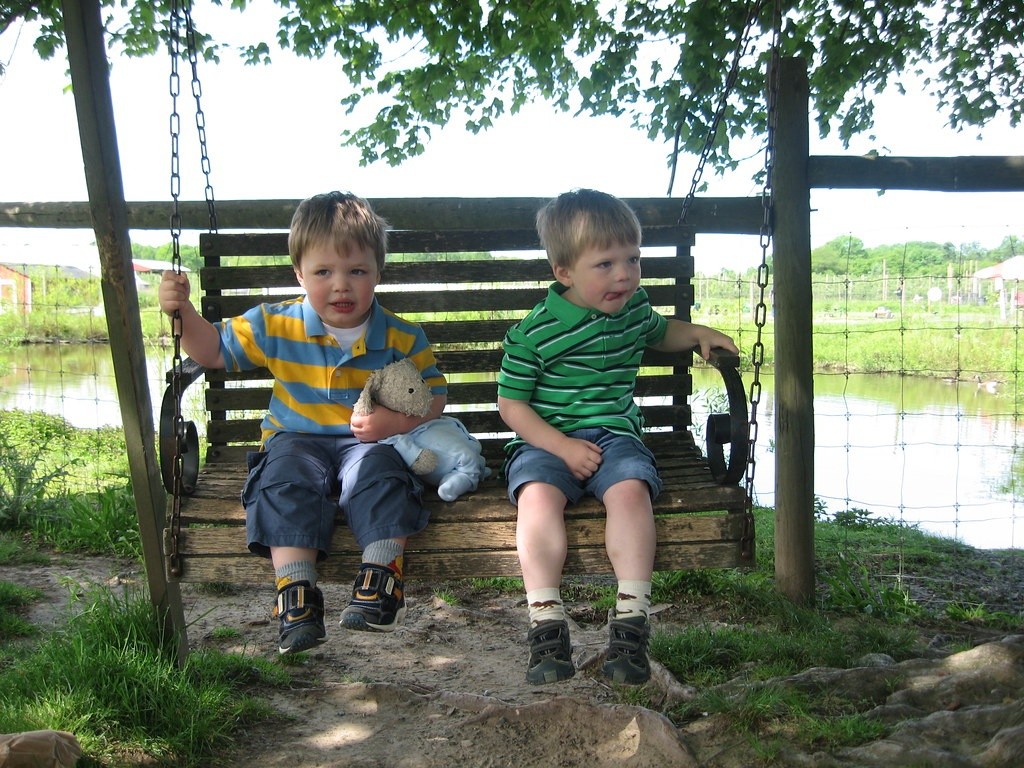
[156,0,780,585]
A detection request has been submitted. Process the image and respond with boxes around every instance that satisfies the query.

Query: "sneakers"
[274,580,327,654]
[339,563,407,633]
[601,607,652,684]
[524,619,575,685]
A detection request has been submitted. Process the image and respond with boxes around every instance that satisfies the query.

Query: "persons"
[158,189,449,656]
[496,185,740,684]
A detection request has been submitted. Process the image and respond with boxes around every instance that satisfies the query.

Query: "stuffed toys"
[351,354,492,502]
